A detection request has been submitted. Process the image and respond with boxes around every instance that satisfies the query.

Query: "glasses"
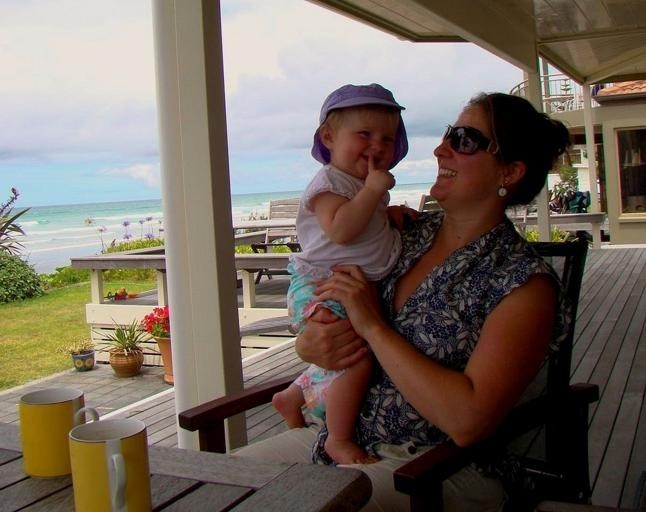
[441,124,501,159]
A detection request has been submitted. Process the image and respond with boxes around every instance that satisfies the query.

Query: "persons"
[231,93,571,512]
[548,181,586,214]
[272,82,419,465]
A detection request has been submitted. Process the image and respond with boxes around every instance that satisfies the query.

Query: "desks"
[0,418,377,511]
[232,218,296,230]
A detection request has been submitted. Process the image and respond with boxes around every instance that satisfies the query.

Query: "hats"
[311,83,408,172]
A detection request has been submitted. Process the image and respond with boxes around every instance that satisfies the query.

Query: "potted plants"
[84,315,152,379]
[55,335,96,373]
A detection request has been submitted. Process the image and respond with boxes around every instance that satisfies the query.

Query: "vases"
[154,335,174,386]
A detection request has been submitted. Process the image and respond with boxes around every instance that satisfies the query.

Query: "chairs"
[413,190,447,216]
[175,229,602,511]
[247,197,306,285]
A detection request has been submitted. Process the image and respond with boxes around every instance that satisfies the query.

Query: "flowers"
[139,304,172,340]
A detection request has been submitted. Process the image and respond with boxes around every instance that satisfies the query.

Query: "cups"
[18,389,100,478]
[69,420,152,511]
[632,147,641,164]
